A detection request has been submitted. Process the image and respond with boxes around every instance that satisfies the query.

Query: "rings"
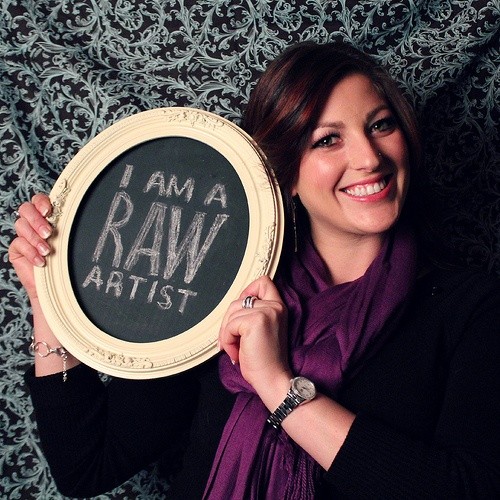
[242,296,258,308]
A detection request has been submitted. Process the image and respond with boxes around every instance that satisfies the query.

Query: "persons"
[8,40,500,500]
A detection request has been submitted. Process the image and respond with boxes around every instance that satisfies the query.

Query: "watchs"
[266,377,317,431]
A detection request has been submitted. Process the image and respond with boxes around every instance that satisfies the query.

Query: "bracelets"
[29,333,70,383]
